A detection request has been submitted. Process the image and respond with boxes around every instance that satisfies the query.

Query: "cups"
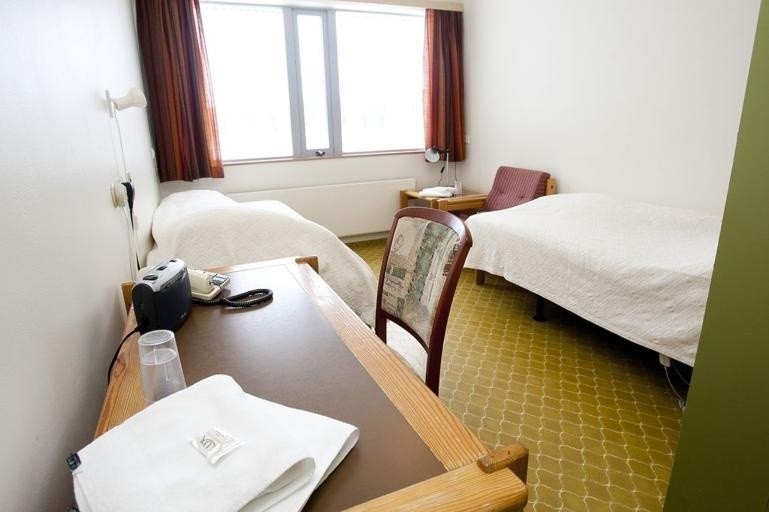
[454,179,462,195]
[136,328,189,409]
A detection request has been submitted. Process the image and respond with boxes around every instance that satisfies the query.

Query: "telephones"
[136,266,231,301]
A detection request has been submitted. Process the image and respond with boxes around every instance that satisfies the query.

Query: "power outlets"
[110,177,122,208]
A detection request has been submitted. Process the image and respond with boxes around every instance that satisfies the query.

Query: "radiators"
[222,178,417,238]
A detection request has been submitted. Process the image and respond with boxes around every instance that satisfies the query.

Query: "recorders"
[131,258,193,336]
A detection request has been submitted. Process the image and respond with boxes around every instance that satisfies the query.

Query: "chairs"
[483,166,557,211]
[377,207,473,391]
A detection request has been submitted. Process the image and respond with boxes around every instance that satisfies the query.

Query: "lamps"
[104,86,147,118]
[424,145,450,186]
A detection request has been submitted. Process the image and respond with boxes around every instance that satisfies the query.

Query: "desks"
[400,186,488,215]
[89,255,527,512]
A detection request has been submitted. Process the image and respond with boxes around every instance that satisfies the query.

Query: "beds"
[460,194,723,377]
